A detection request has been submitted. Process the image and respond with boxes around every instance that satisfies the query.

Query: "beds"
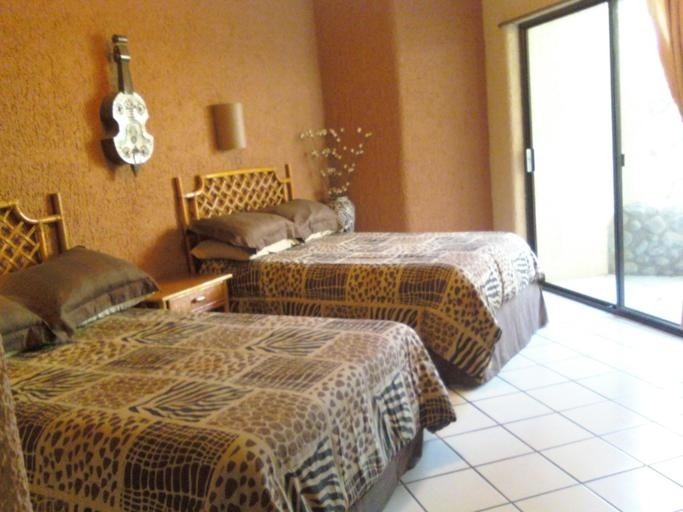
[0,192,455,512]
[175,166,550,382]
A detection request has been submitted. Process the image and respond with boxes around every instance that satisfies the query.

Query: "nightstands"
[141,273,233,314]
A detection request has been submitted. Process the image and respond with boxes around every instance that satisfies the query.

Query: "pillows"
[0,246,157,357]
[189,198,338,262]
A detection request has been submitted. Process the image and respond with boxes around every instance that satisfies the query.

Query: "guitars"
[100,34,154,165]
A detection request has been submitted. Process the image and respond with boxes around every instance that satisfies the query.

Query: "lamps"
[214,103,247,151]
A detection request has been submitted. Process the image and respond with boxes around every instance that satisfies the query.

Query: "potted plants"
[300,126,373,232]
